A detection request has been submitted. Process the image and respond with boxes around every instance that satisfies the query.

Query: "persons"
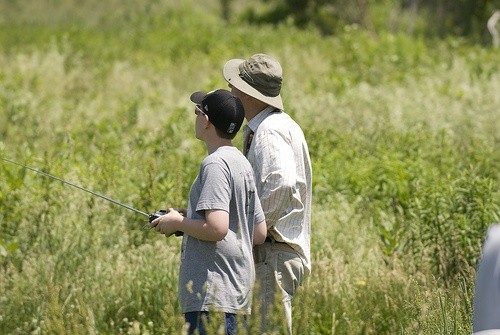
[222,54,311,335]
[151,89,267,335]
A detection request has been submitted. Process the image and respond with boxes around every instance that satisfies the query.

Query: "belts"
[265,234,285,244]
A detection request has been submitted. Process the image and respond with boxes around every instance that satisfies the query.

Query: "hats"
[223,53,284,110]
[190,89,245,134]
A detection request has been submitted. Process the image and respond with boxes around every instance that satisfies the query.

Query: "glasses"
[228,84,240,93]
[195,104,205,118]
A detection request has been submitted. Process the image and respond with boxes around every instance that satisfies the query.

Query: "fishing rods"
[0,157,183,236]
